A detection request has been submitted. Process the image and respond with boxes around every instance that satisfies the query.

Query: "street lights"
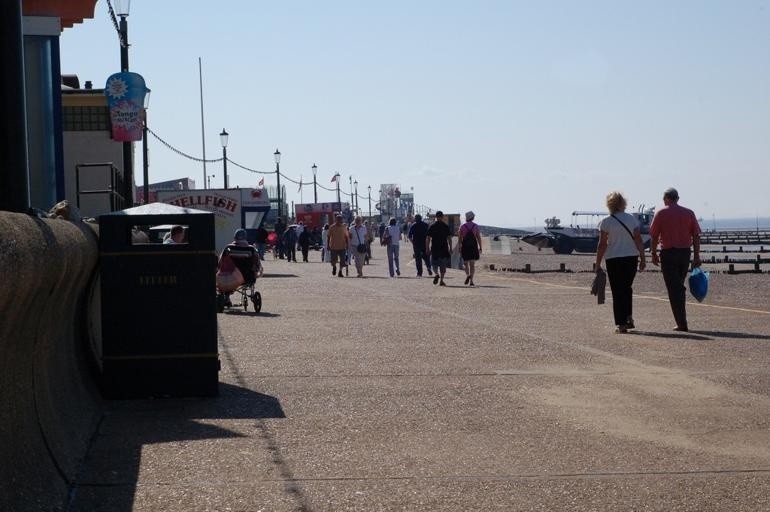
[350,178,434,224]
[335,171,342,203]
[112,0,136,212]
[272,148,282,217]
[218,126,230,189]
[311,161,318,203]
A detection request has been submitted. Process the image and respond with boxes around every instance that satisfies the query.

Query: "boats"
[524,202,657,258]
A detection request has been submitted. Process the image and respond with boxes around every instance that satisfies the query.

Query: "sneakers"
[434,275,439,284]
[440,282,445,286]
[465,276,469,284]
[615,325,627,333]
[626,318,633,328]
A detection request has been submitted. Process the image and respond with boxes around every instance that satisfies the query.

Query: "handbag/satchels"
[381,228,392,246]
[216,253,244,289]
[358,244,366,253]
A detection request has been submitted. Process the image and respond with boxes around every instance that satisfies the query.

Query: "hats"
[234,229,247,239]
[466,211,475,222]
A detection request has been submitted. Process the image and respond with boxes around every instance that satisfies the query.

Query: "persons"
[218,229,263,305]
[648,188,701,331]
[163,225,184,245]
[595,192,646,334]
[255,211,483,286]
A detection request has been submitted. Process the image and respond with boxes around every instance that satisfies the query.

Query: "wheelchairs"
[217,246,266,317]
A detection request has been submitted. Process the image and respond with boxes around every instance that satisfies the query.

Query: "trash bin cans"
[98,202,219,399]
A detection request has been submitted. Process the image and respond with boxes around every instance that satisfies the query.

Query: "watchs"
[651,251,656,255]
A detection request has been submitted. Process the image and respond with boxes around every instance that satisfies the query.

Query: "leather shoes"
[673,327,688,331]
